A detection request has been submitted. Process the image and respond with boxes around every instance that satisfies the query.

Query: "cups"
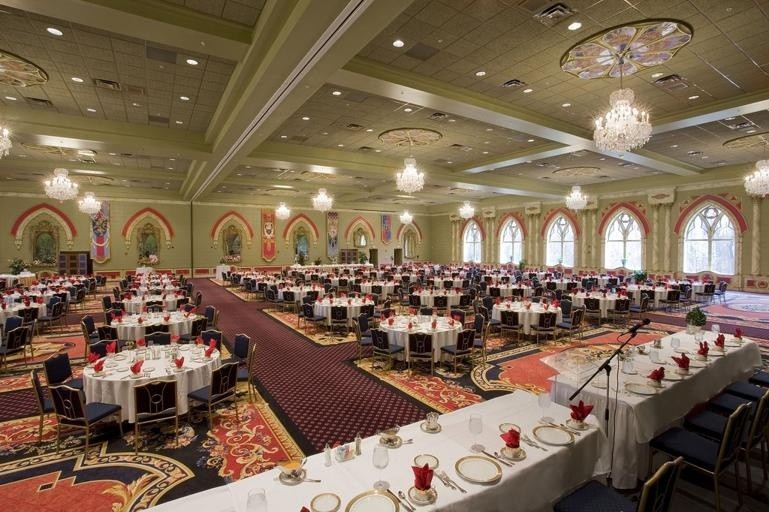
[277,459,302,476]
[377,424,400,444]
[415,484,432,501]
[505,444,520,457]
[247,487,266,511]
[572,417,585,428]
[131,311,136,320]
[148,307,159,318]
[337,445,354,461]
[425,412,439,430]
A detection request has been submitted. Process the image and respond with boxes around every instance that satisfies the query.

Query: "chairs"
[82,270,222,356]
[225,265,729,375]
[134,381,177,451]
[2,274,107,370]
[28,352,123,457]
[550,365,769,512]
[188,334,258,434]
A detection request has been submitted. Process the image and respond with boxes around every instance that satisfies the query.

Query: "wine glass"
[537,392,551,420]
[372,444,390,492]
[469,414,483,449]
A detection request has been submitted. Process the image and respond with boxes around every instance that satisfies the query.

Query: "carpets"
[1,375,325,512]
[645,287,769,332]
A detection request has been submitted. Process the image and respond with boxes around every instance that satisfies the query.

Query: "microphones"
[624,314,651,337]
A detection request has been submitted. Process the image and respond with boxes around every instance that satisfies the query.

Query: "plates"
[592,324,745,395]
[533,426,574,445]
[500,447,526,461]
[310,492,341,512]
[380,436,403,448]
[279,470,307,483]
[345,490,400,512]
[420,422,441,433]
[409,486,438,504]
[567,419,589,430]
[414,454,439,470]
[498,422,521,435]
[89,341,217,381]
[455,456,501,482]
[387,315,460,332]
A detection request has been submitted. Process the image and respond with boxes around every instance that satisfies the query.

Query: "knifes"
[521,435,547,451]
[439,471,468,495]
[480,450,512,467]
[549,421,581,435]
[387,489,412,512]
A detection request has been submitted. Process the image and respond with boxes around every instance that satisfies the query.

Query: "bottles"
[323,444,332,467]
[355,432,361,455]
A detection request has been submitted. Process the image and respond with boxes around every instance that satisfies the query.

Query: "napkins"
[173,356,185,368]
[131,358,145,374]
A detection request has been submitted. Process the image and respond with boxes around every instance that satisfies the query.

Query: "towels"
[733,326,744,339]
[410,463,434,491]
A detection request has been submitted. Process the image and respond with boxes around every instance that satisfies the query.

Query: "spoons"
[494,451,515,465]
[398,490,416,511]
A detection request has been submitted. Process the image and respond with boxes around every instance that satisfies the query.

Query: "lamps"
[591,55,654,156]
[40,168,80,203]
[564,178,588,213]
[0,127,13,158]
[742,142,769,199]
[78,191,103,216]
[273,151,477,227]
